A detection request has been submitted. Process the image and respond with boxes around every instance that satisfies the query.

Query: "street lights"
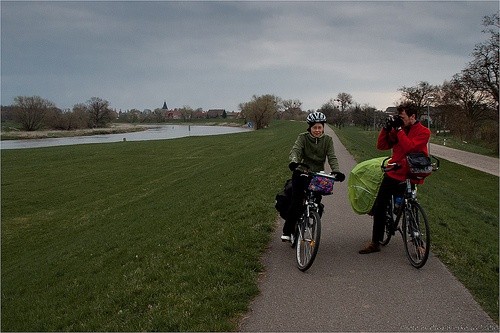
[427,97,434,156]
[373,110,378,138]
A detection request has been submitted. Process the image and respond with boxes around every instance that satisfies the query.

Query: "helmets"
[307,112,326,123]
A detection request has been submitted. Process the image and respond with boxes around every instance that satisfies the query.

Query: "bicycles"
[281,167,338,271]
[378,154,438,269]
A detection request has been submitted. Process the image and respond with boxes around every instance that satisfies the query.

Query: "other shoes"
[359,242,381,254]
[281,235,292,241]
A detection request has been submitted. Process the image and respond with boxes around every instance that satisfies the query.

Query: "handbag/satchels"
[407,151,432,169]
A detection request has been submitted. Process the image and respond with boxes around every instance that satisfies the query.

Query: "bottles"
[394,194,404,208]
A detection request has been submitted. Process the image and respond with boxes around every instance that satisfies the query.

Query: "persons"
[358,103,430,254]
[280,112,346,240]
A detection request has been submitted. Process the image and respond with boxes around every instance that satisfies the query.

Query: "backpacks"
[275,179,292,220]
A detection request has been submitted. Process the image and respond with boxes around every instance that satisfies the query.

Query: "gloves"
[391,118,402,128]
[289,162,298,170]
[331,172,345,182]
[386,119,391,131]
